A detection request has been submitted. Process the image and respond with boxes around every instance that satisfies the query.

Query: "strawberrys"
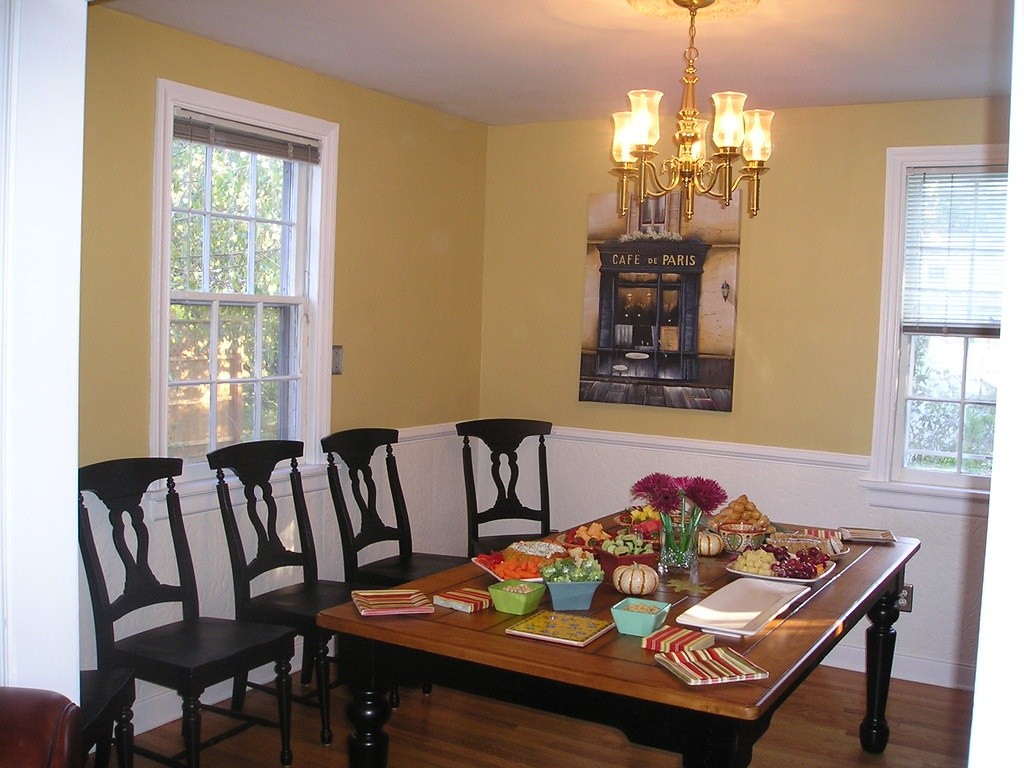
[563,530,617,546]
[619,514,671,540]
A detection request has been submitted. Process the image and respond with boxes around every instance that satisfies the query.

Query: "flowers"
[629,476,727,547]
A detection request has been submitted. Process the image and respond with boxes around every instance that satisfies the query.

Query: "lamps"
[610,1,776,221]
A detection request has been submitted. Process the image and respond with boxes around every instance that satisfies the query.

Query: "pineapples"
[631,504,661,522]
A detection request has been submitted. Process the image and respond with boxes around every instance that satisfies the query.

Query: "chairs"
[455,417,559,557]
[319,427,466,706]
[77,457,300,766]
[207,439,403,744]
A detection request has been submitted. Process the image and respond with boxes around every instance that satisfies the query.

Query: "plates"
[725,559,836,585]
[694,515,776,534]
[676,577,812,638]
[653,648,769,686]
[351,590,434,616]
[828,545,851,561]
[471,557,543,583]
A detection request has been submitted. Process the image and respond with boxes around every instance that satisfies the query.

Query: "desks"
[314,506,923,766]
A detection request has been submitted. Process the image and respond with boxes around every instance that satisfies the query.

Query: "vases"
[657,513,701,576]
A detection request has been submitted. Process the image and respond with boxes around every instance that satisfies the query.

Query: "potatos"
[715,495,777,534]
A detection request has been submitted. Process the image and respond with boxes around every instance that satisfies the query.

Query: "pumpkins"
[697,532,723,556]
[613,564,659,594]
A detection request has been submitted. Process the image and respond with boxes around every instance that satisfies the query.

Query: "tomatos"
[476,552,505,568]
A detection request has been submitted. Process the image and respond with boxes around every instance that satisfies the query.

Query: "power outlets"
[897,583,914,612]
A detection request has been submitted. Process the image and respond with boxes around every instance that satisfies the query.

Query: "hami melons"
[575,523,611,541]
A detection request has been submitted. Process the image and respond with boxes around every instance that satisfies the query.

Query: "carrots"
[493,560,545,580]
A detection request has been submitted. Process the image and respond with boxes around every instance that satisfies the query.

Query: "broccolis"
[541,559,604,583]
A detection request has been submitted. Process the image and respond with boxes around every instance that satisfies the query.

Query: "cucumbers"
[603,534,655,555]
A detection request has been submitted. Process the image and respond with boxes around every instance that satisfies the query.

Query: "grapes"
[729,544,830,579]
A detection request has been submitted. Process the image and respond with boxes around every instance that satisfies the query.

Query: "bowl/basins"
[613,516,633,527]
[611,597,672,637]
[616,529,679,549]
[488,579,546,616]
[592,546,660,584]
[718,522,766,555]
[556,531,612,559]
[540,570,602,611]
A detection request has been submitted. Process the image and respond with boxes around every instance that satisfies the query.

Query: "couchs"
[0,687,83,768]
